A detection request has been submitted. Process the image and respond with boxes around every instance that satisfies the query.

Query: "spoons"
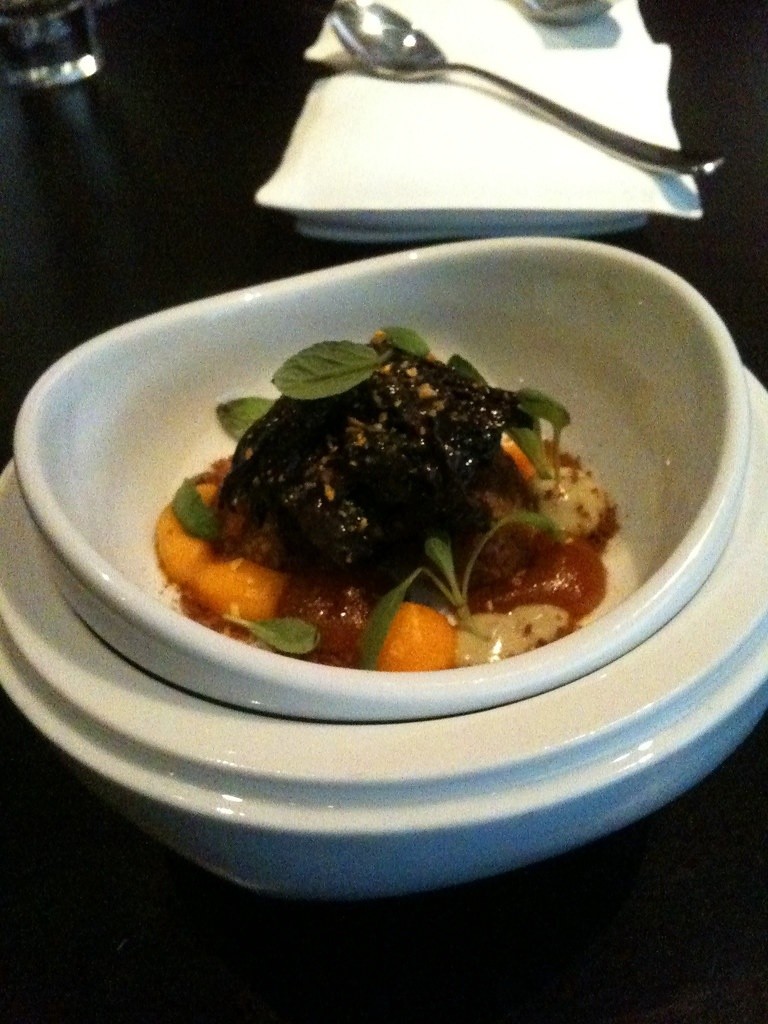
[331,0,726,176]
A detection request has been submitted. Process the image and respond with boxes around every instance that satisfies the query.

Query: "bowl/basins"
[16,236,755,716]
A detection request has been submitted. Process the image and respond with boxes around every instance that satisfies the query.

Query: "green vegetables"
[172,325,572,671]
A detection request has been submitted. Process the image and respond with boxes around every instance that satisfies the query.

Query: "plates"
[302,0,654,72]
[256,42,706,243]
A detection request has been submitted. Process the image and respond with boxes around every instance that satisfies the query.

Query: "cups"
[0,0,106,87]
